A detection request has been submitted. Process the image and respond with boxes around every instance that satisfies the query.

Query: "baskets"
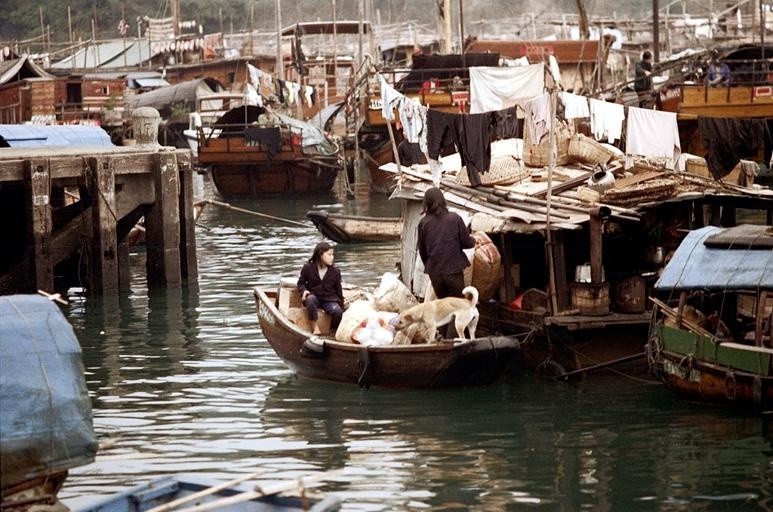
[567,132,614,166]
[737,293,773,319]
[522,118,574,166]
[456,155,530,186]
[686,158,710,178]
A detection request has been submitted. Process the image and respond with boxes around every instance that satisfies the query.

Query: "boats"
[307,200,402,247]
[0,291,344,512]
[1,123,206,254]
[639,215,772,401]
[252,281,524,391]
[378,88,772,381]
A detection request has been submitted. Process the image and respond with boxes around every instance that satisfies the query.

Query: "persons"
[452,76,464,92]
[257,104,278,128]
[703,48,731,87]
[633,51,653,107]
[417,74,440,94]
[297,242,350,337]
[396,131,422,167]
[416,187,475,343]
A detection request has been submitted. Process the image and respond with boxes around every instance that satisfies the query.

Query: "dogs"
[394,285,479,344]
